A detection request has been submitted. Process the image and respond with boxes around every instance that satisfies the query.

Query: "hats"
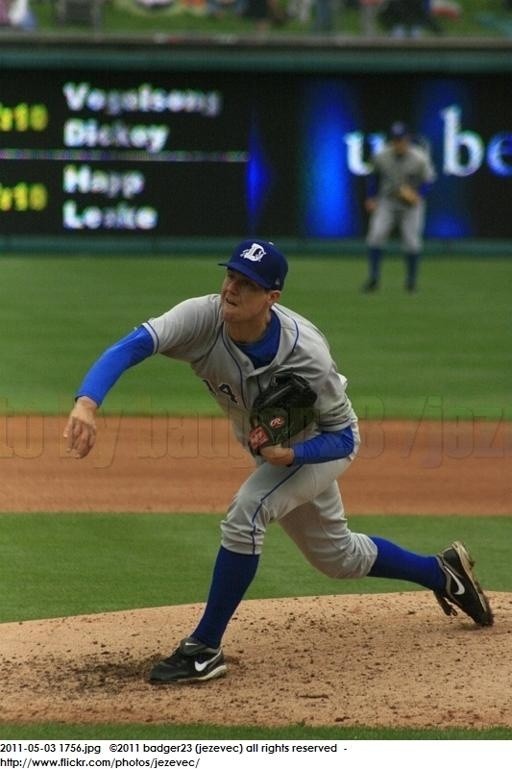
[216,237,290,291]
[385,120,407,140]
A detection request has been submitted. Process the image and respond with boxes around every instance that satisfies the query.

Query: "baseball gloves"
[398,182,421,208]
[248,375,317,457]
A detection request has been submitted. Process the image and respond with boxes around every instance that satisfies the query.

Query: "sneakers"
[433,538,495,629]
[149,634,231,687]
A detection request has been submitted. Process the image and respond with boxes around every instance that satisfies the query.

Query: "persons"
[359,120,439,293]
[61,237,496,685]
